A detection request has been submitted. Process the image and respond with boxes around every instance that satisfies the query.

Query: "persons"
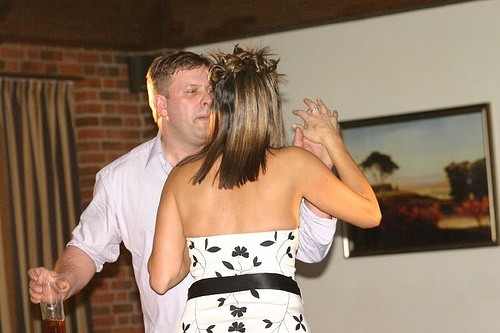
[28,48,342,333]
[146,42,382,332]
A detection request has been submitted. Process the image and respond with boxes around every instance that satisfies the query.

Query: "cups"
[37,281,66,333]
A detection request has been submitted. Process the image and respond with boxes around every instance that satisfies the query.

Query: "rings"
[310,105,319,113]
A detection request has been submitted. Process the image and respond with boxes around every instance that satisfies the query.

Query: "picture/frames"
[332,102,500,260]
